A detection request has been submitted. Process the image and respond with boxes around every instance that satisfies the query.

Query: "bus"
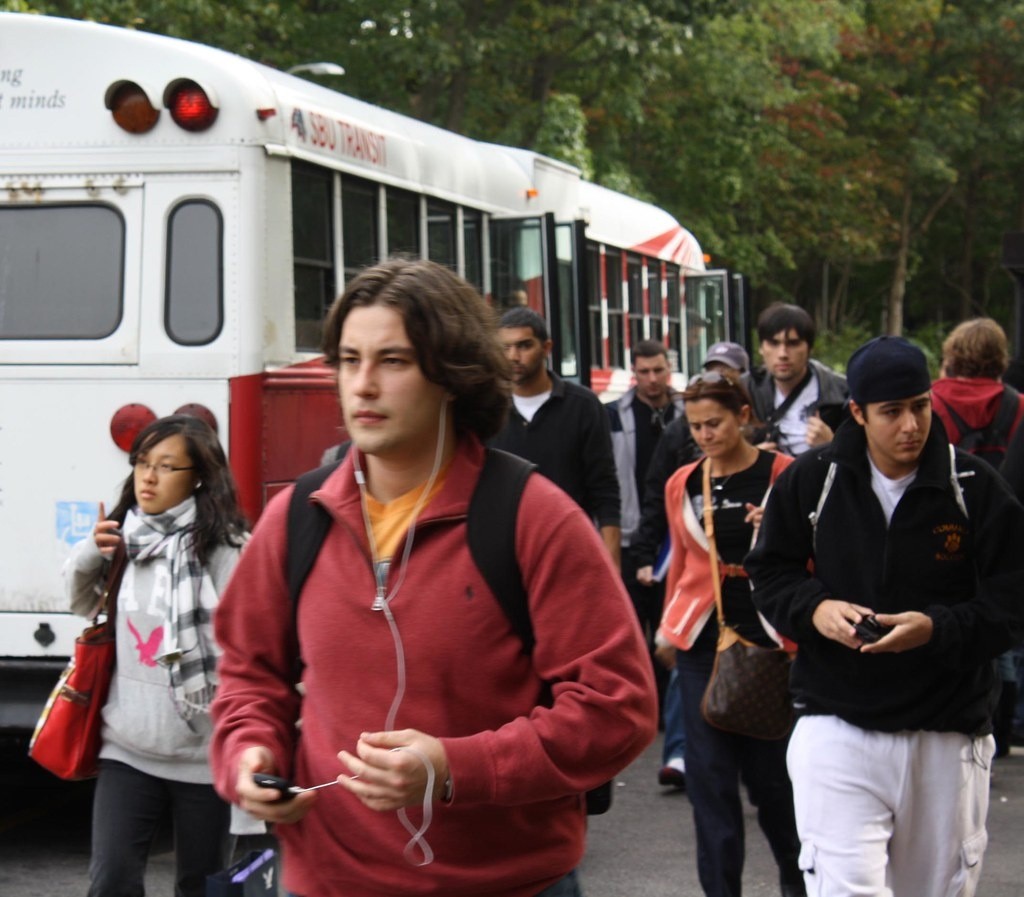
[0,10,745,729]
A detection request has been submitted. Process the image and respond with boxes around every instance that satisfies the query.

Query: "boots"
[995,680,1016,758]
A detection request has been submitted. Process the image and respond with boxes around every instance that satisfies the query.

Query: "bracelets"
[443,777,453,803]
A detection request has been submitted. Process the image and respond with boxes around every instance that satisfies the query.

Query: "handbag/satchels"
[698,624,798,741]
[25,620,115,783]
[204,828,278,897]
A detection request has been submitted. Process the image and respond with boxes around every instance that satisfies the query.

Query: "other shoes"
[656,753,688,789]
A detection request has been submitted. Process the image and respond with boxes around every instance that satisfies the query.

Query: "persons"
[655,369,816,897]
[210,260,657,897]
[749,303,848,458]
[928,317,1024,488]
[743,337,1024,897]
[610,340,688,590]
[489,307,622,575]
[69,413,253,897]
[629,341,750,787]
[490,271,527,307]
[687,308,703,350]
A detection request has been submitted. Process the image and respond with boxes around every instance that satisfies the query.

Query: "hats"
[700,340,748,373]
[844,333,932,406]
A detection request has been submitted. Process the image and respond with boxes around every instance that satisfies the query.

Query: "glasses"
[129,452,201,477]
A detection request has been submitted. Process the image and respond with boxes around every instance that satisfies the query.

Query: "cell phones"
[252,772,296,800]
[854,615,887,642]
